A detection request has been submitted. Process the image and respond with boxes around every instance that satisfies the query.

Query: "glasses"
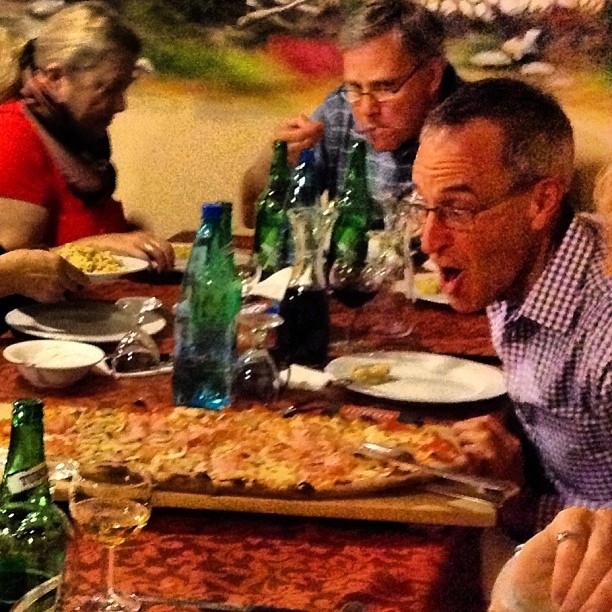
[336,68,419,101]
[409,176,568,231]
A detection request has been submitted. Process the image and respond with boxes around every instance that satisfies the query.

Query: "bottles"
[281,150,317,214]
[0,398,72,612]
[276,204,338,369]
[328,137,381,294]
[168,201,239,411]
[245,139,292,283]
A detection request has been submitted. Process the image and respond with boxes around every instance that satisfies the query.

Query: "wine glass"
[67,462,151,612]
[228,313,283,405]
[112,296,163,371]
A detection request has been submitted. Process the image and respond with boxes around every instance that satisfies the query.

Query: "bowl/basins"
[3,341,105,392]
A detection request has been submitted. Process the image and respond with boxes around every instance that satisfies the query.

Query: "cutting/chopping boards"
[50,419,495,527]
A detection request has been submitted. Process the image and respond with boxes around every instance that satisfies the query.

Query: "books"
[167,230,255,275]
[410,272,450,293]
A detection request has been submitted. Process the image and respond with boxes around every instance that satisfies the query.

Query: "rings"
[556,530,585,541]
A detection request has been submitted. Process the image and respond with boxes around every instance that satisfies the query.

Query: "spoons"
[319,124,381,136]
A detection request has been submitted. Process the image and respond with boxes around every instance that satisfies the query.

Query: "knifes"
[354,443,509,492]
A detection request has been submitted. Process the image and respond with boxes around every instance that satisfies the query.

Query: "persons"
[488,505,611,612]
[240,1,466,230]
[0,249,90,304]
[1,1,177,273]
[410,77,612,508]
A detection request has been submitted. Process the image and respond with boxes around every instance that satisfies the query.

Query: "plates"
[322,349,506,402]
[7,303,166,341]
[83,251,149,277]
